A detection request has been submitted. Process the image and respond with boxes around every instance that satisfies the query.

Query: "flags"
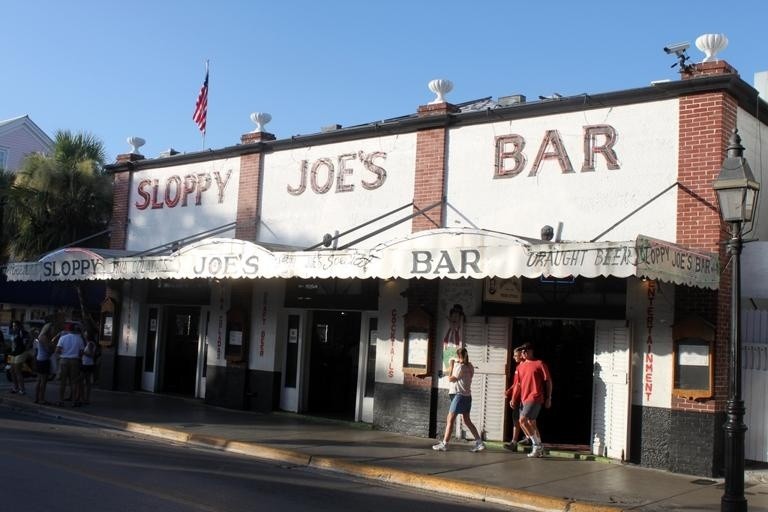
[193,70,208,135]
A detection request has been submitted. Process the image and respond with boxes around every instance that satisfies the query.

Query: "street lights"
[709,128,762,510]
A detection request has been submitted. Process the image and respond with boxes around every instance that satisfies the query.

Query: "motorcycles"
[4,335,57,382]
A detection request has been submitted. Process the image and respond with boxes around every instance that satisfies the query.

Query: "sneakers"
[503,442,518,452]
[472,443,485,451]
[519,438,545,458]
[432,444,448,451]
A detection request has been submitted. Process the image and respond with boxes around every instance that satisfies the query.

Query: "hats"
[517,343,533,350]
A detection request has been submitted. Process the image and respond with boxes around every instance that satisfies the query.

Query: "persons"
[432,346,486,454]
[1,315,98,408]
[504,346,541,451]
[510,343,553,457]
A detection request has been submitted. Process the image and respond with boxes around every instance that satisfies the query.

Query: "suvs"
[22,318,87,350]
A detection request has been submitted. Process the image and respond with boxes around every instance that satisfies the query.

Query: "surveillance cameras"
[664,43,689,57]
